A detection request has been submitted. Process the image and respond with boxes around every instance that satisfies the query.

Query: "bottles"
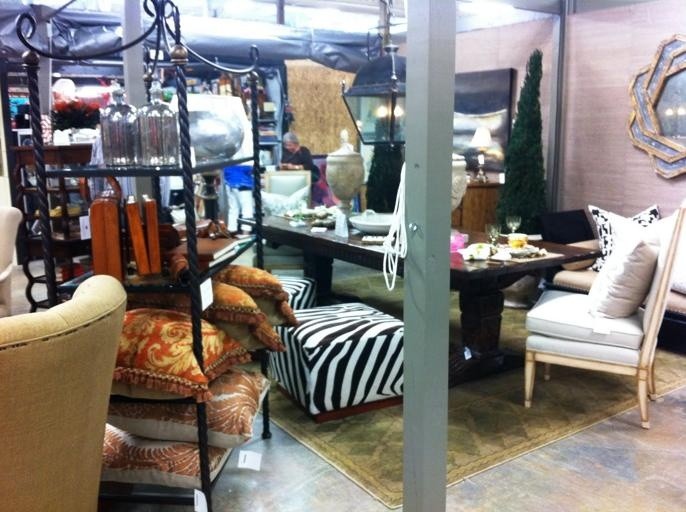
[450,151,469,213]
[194,66,268,118]
[101,89,140,167]
[139,83,181,166]
[325,129,364,209]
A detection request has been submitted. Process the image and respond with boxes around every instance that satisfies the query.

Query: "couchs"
[528,209,686,357]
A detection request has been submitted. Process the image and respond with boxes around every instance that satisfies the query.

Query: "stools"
[267,304,404,423]
[250,273,317,361]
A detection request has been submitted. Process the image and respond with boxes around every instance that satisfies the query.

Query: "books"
[90,194,251,282]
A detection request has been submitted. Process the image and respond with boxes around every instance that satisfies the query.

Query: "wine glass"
[485,216,528,251]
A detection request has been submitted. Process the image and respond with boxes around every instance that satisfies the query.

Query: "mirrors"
[626,33,686,179]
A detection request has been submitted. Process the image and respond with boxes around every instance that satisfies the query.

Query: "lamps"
[340,38,406,145]
[469,126,494,185]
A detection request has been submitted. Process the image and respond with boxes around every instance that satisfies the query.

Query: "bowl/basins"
[348,208,395,235]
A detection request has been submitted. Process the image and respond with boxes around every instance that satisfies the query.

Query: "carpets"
[257,273,686,509]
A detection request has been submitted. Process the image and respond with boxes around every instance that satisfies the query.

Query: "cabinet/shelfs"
[451,183,503,233]
[17,0,271,512]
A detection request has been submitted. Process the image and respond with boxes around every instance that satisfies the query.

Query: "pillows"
[587,233,660,320]
[586,198,686,295]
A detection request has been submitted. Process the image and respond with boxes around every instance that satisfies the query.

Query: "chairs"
[523,206,686,430]
[0,207,127,512]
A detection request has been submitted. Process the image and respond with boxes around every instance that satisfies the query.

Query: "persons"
[221,162,255,236]
[275,132,320,181]
[89,104,170,207]
